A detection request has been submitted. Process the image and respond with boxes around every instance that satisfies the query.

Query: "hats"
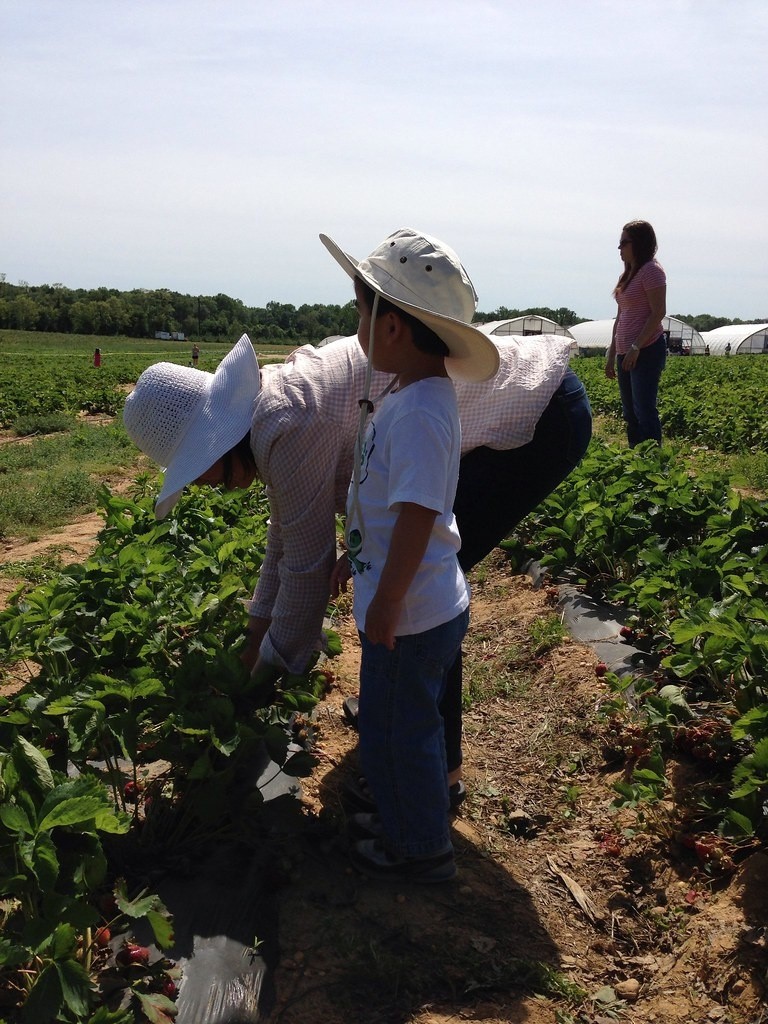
[320,229,500,385]
[122,334,261,522]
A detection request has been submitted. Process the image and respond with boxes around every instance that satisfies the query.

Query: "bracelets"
[632,344,639,351]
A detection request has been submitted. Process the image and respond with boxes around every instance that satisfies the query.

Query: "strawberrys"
[330,676,335,682]
[620,626,632,635]
[96,927,110,945]
[125,782,141,795]
[117,945,141,962]
[151,975,175,995]
[596,665,606,675]
[140,947,150,960]
[324,671,330,676]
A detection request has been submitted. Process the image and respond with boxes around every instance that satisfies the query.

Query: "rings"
[629,362,632,365]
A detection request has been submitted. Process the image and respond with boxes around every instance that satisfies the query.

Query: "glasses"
[619,238,633,246]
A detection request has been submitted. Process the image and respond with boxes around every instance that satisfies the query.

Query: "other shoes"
[340,811,459,884]
[346,766,467,809]
[344,700,360,730]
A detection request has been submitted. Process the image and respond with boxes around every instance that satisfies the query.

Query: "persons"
[93,348,102,367]
[666,345,690,356]
[705,345,710,356]
[604,220,667,451]
[125,231,593,885]
[725,343,731,355]
